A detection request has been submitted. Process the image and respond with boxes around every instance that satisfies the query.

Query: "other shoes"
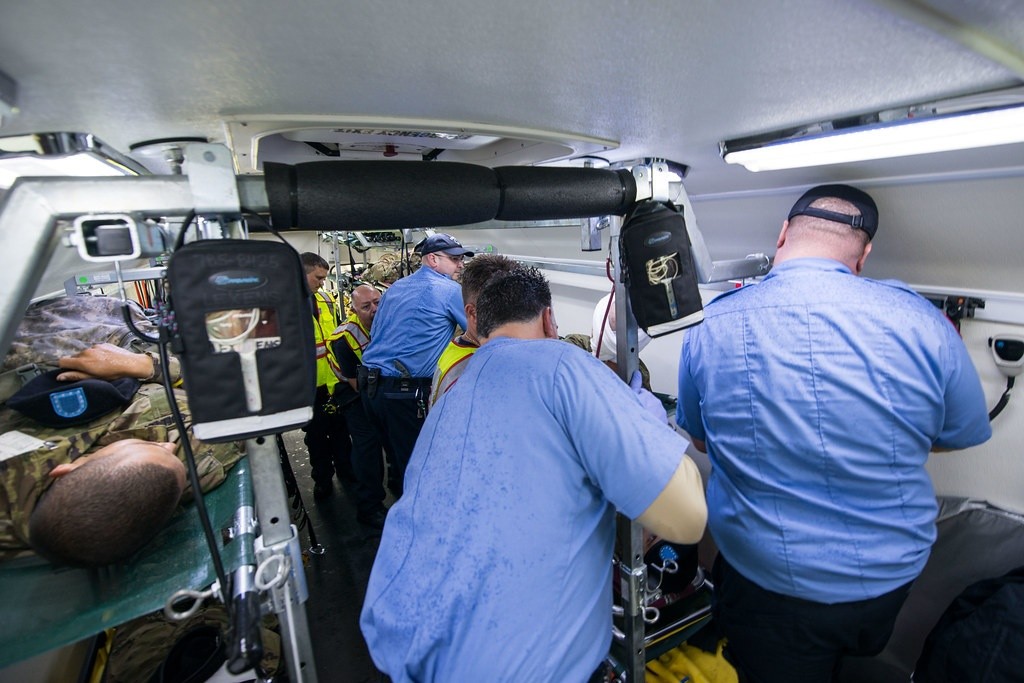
[313,481,334,503]
[356,502,389,530]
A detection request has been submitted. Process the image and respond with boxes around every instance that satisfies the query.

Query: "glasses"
[432,252,465,263]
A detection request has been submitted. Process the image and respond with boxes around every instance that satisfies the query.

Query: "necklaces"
[464,331,480,348]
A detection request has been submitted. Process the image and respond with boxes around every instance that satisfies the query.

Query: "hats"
[787,184,879,242]
[6,367,143,429]
[421,233,474,257]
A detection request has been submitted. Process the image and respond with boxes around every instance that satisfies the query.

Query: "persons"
[301,252,337,503]
[675,184,992,683]
[428,256,520,415]
[0,343,249,564]
[589,291,654,397]
[324,284,382,505]
[359,265,709,683]
[352,232,476,531]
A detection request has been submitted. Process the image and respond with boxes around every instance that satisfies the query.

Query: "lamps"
[718,85,1024,173]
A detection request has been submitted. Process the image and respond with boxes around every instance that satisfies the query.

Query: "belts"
[379,376,432,386]
